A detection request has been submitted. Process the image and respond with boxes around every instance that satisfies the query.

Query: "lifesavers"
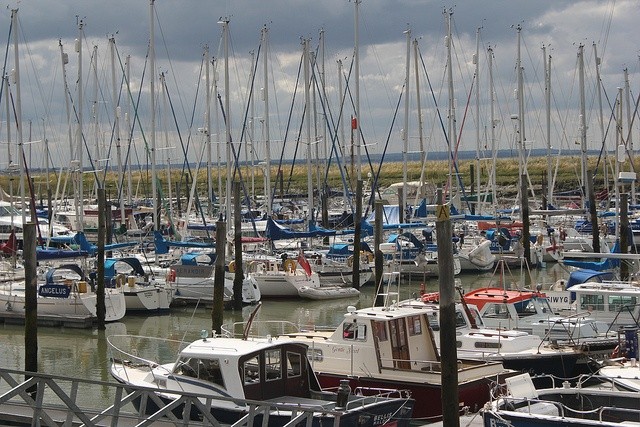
[365,251,373,262]
[115,274,126,287]
[348,254,355,267]
[422,291,440,301]
[248,260,259,274]
[229,260,235,273]
[284,259,296,272]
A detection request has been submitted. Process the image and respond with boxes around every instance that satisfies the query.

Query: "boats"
[105,333,417,424]
[379,292,585,394]
[430,284,628,369]
[237,303,524,423]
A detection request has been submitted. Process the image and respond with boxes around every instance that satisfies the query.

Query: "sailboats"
[1,0,639,335]
